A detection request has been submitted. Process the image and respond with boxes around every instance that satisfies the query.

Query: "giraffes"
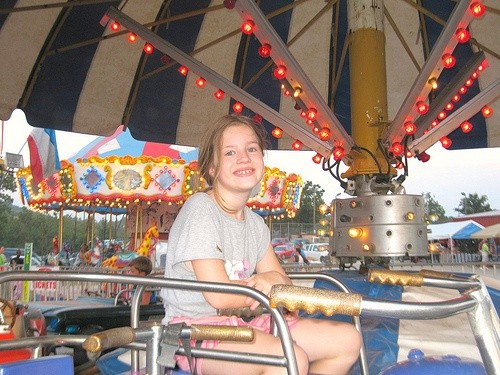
[97,226,159,295]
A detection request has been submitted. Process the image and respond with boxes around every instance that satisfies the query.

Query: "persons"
[480,240,492,271]
[0,245,10,299]
[127,256,157,305]
[10,250,25,291]
[435,242,442,263]
[160,113,363,375]
[290,241,311,272]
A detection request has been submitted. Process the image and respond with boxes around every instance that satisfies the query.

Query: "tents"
[426,219,500,263]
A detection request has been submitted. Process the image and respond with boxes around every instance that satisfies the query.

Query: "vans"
[300,242,330,267]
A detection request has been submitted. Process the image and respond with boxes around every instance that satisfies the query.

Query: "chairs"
[133,273,371,375]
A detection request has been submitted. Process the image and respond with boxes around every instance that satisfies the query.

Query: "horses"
[82,238,103,266]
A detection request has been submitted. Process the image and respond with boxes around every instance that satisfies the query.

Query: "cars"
[273,244,295,260]
[271,237,289,248]
[4,247,41,262]
[291,239,310,252]
